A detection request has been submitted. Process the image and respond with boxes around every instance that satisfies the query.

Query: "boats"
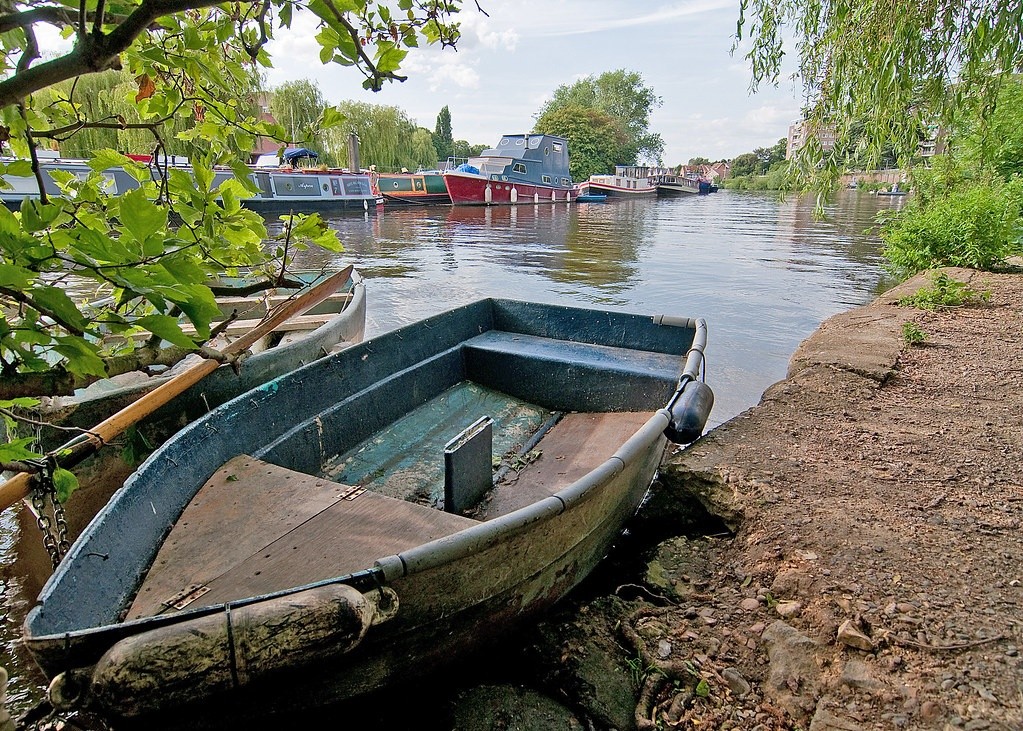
[441,133,580,205]
[588,164,656,198]
[656,173,700,194]
[0,266,367,552]
[22,298,714,725]
[576,191,607,202]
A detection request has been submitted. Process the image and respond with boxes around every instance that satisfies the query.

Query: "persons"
[401,167,409,174]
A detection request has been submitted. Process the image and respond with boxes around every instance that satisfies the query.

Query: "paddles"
[0,263,359,511]
